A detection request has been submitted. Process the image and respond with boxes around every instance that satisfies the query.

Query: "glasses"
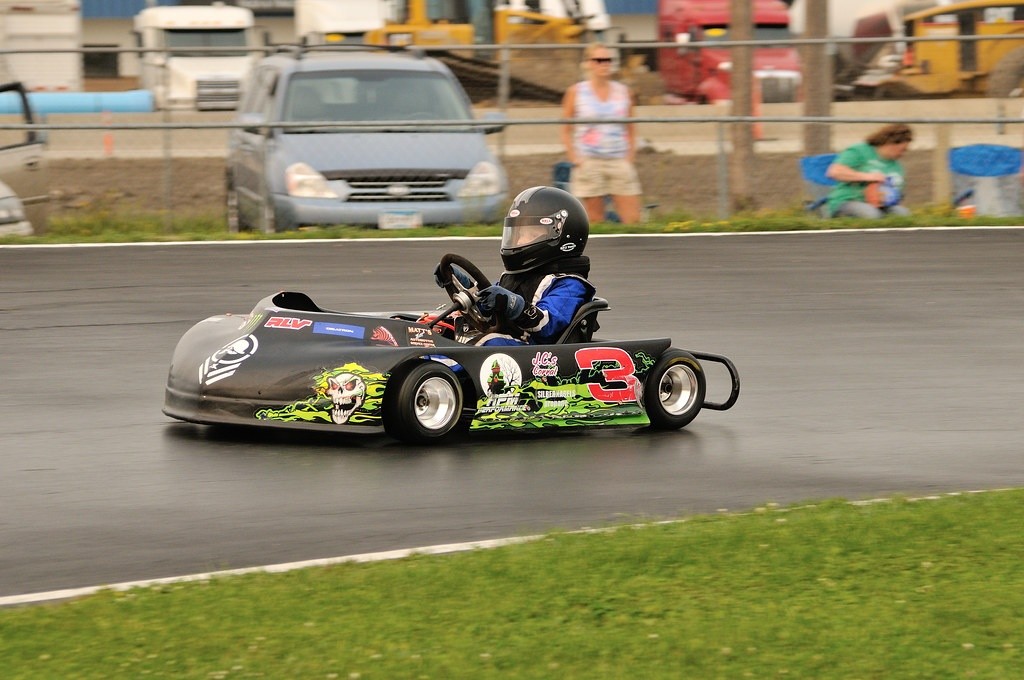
[586,58,611,63]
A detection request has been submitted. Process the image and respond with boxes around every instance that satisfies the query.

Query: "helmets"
[500,186,590,275]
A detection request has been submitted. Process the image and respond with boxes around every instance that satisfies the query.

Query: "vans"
[223,48,511,236]
[133,2,258,113]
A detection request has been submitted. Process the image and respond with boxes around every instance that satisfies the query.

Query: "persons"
[562,43,642,225]
[826,124,912,222]
[415,185,597,372]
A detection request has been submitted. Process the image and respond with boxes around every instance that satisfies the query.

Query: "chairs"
[947,142,1023,220]
[801,154,837,187]
[554,295,610,346]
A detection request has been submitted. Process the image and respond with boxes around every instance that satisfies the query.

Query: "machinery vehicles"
[312,0,1024,99]
[657,2,813,106]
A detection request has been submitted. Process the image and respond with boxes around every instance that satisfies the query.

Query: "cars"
[0,80,49,245]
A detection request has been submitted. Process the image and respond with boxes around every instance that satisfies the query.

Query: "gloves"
[434,261,471,289]
[475,286,525,319]
